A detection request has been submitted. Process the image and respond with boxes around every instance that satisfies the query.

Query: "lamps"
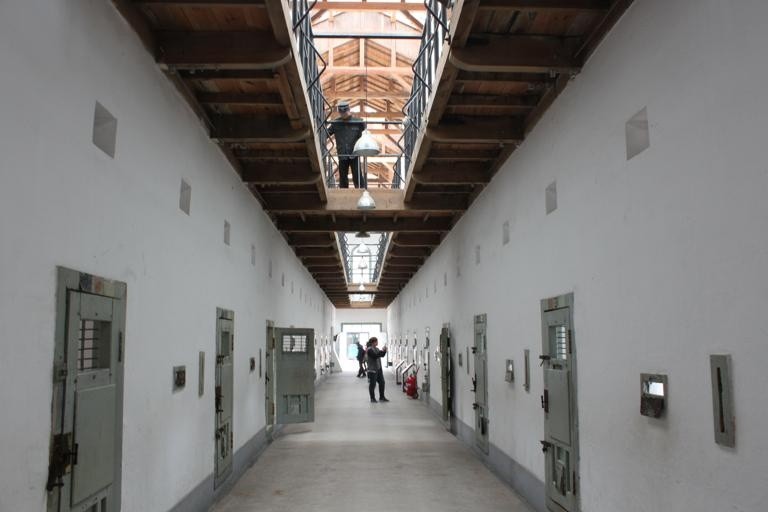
[352,37,381,211]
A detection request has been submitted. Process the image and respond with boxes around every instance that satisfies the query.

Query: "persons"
[355,339,366,378]
[364,336,391,403]
[326,101,367,190]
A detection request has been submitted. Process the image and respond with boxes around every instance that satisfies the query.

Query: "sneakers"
[370,396,389,403]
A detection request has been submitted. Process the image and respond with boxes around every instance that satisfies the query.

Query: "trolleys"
[404,371,418,400]
